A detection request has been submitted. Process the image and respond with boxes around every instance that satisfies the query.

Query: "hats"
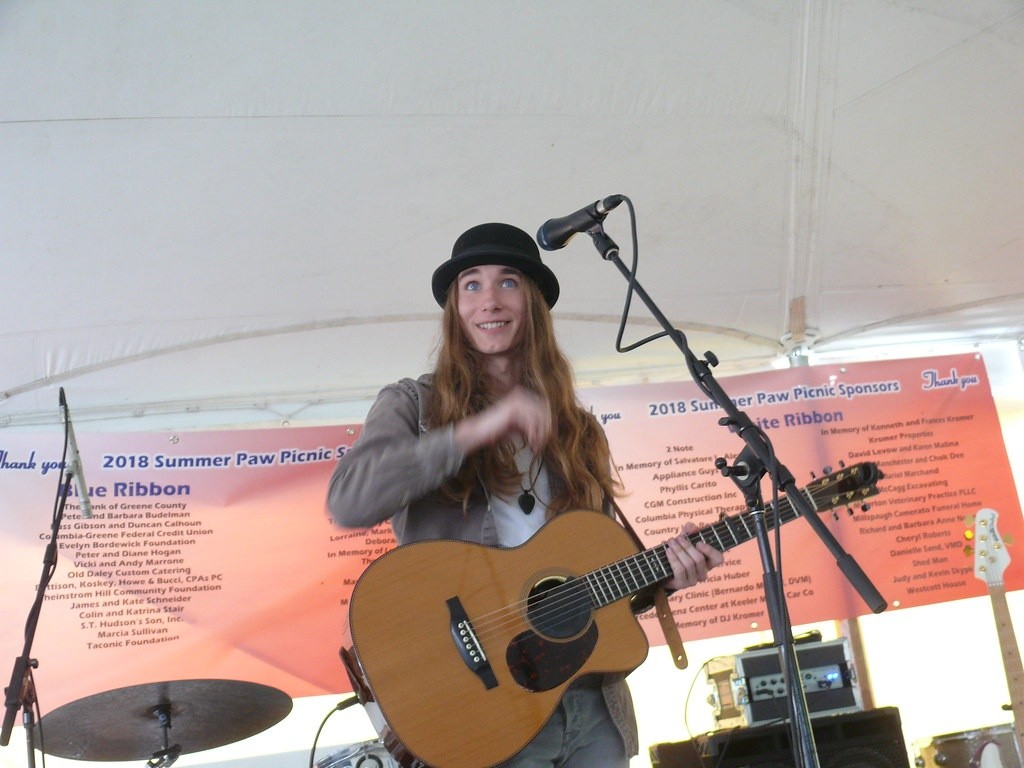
[432,223,560,312]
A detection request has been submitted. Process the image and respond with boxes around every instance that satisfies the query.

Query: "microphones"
[59,395,93,519]
[537,193,624,251]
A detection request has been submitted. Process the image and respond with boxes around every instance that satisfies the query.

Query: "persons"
[327,223,726,768]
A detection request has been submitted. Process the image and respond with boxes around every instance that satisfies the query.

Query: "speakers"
[650,706,909,768]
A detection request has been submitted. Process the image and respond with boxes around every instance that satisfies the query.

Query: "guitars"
[958,505,1024,768]
[336,453,887,768]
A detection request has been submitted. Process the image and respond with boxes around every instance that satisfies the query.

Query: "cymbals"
[30,676,296,763]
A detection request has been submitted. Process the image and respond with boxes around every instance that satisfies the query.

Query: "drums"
[315,737,403,768]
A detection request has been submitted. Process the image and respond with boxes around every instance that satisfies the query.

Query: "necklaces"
[511,451,544,515]
